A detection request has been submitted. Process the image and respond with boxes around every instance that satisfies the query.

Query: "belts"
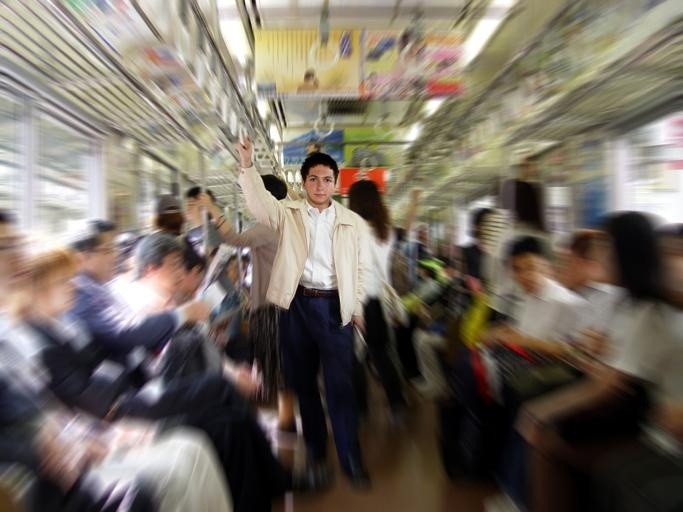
[293,284,338,297]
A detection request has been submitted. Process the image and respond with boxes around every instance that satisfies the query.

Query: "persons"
[0,174,395,507]
[394,178,681,507]
[236,137,369,497]
